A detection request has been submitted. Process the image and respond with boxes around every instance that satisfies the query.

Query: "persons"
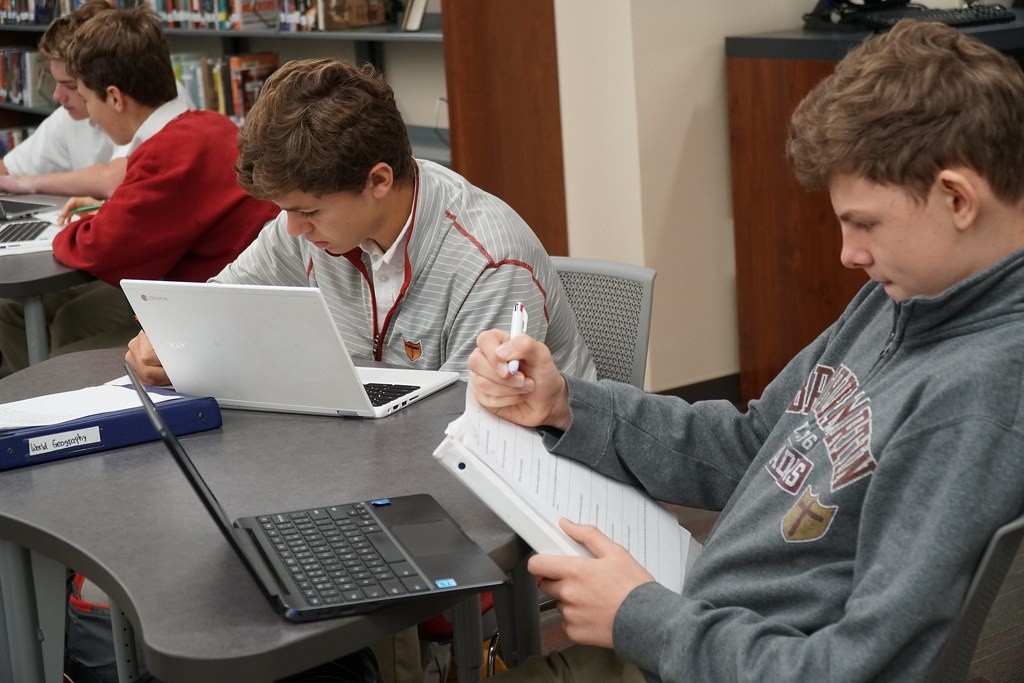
[124,60,595,682]
[0,0,197,380]
[466,19,1024,682]
[47,0,283,361]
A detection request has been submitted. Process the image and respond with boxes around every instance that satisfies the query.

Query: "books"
[0,44,280,122]
[0,0,431,32]
[0,125,34,160]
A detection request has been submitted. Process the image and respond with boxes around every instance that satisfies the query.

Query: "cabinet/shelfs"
[0,0,568,253]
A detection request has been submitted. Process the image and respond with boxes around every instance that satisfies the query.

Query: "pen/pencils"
[69,204,100,216]
[506,300,528,374]
[134,316,143,329]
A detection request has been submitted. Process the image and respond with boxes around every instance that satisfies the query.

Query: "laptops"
[0,199,63,249]
[124,364,512,624]
[118,278,461,418]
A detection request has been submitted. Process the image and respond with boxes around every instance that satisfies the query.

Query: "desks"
[724,17,1024,407]
[0,191,96,367]
[0,323,544,683]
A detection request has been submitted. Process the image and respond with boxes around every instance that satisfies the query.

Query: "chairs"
[480,255,1024,683]
